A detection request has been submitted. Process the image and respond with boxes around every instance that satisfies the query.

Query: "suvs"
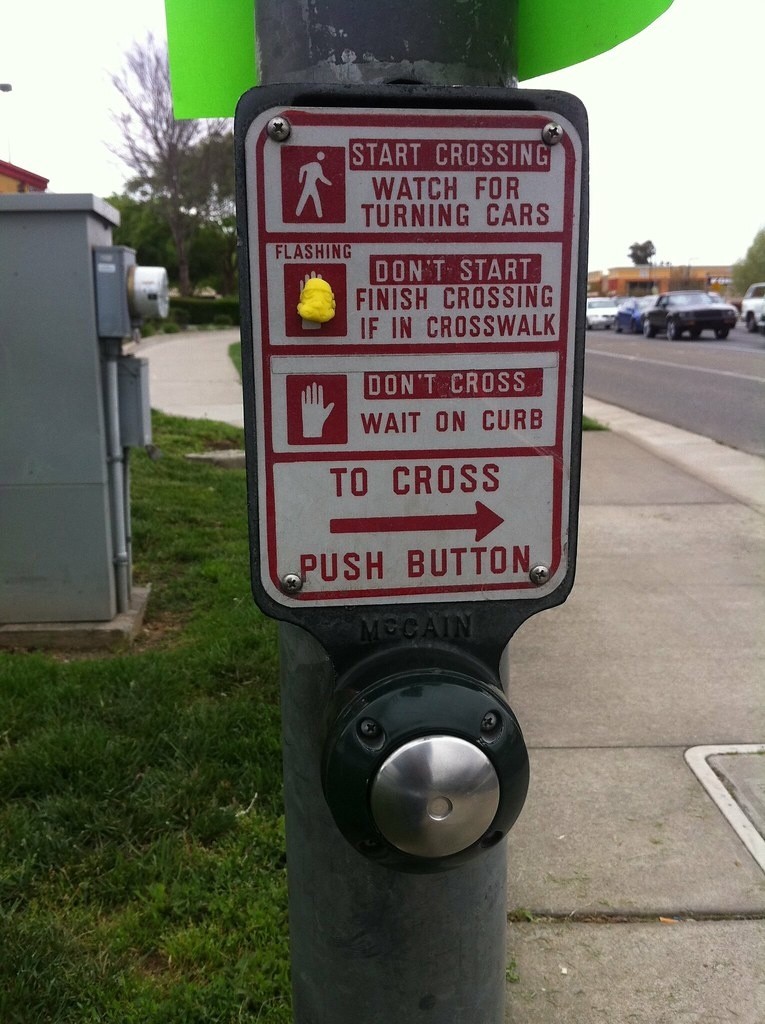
[739,282,765,331]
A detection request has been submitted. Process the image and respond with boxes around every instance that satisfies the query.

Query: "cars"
[585,297,616,330]
[615,298,643,334]
[641,290,738,341]
